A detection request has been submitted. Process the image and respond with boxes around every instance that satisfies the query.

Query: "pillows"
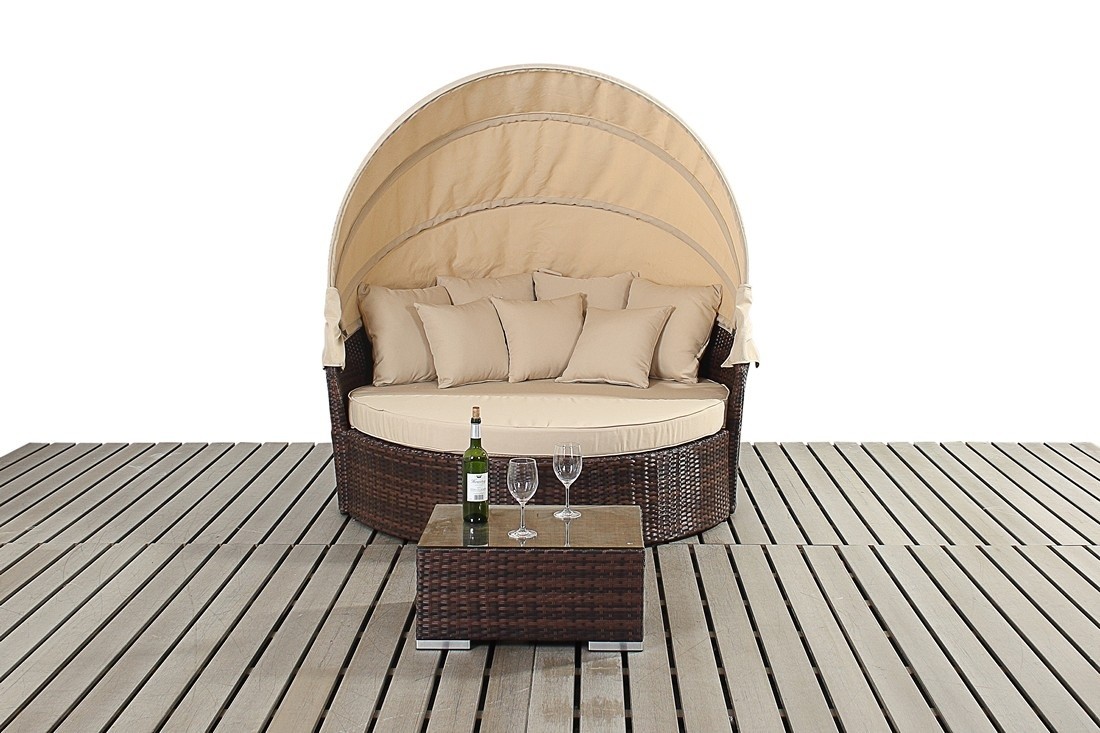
[555,305,676,387]
[626,278,723,384]
[491,293,587,384]
[412,297,509,387]
[358,279,454,387]
[437,271,535,303]
[532,267,640,311]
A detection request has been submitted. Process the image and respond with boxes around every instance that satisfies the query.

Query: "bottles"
[462,406,490,525]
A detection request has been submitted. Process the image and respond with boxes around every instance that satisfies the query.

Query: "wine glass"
[505,457,538,540]
[552,441,582,521]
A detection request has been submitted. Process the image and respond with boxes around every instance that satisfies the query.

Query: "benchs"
[323,60,761,547]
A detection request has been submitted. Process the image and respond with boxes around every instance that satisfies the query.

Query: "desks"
[415,504,646,652]
